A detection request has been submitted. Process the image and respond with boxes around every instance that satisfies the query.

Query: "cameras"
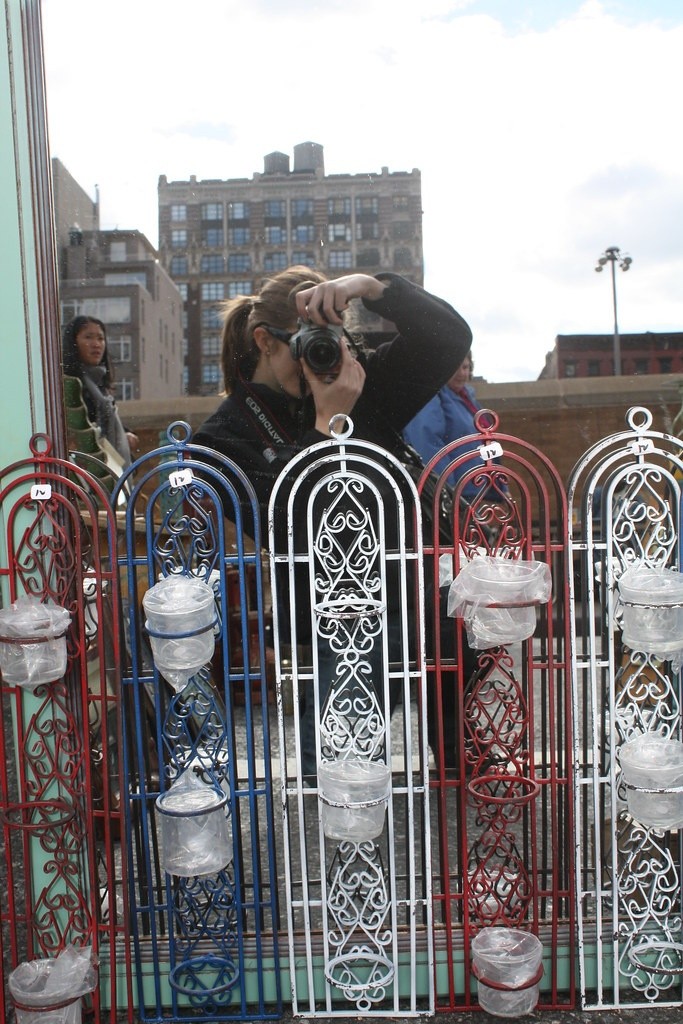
[290,304,343,372]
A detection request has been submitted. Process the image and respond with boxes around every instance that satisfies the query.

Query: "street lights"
[595,247,632,375]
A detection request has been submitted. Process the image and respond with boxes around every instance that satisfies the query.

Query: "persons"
[60,315,140,509]
[186,266,502,779]
[397,346,513,511]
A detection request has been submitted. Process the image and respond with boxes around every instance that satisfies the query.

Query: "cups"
[620,743,683,824]
[170,749,230,818]
[321,717,384,763]
[9,959,82,1024]
[0,605,69,684]
[162,790,232,877]
[597,708,652,776]
[621,575,683,652]
[143,585,215,670]
[158,566,222,635]
[599,555,661,627]
[319,761,389,841]
[468,564,536,643]
[461,548,517,568]
[472,930,541,1017]
[457,873,516,923]
